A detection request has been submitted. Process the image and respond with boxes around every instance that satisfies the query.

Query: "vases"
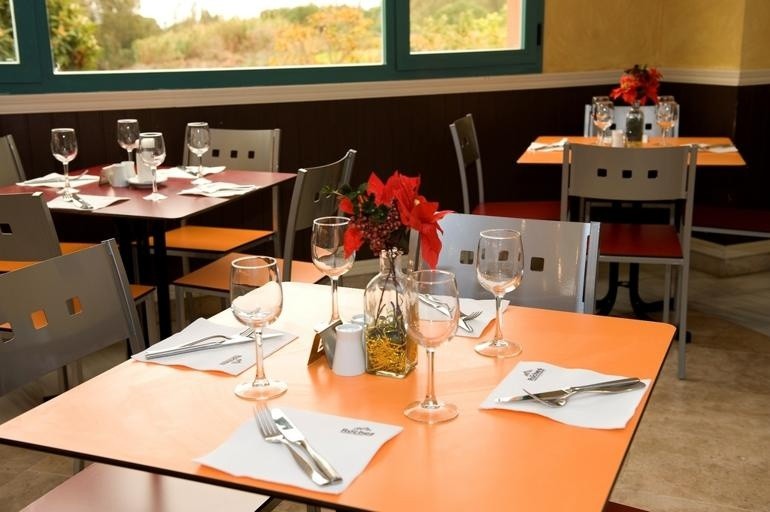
[625,104,646,146]
[364,250,419,379]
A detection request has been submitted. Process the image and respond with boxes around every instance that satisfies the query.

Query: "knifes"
[492,377,640,403]
[265,402,343,485]
[176,165,199,176]
[146,333,286,360]
[414,294,473,333]
[71,193,93,209]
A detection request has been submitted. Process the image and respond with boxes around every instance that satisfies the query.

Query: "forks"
[251,400,330,487]
[425,294,484,321]
[522,380,646,408]
[25,170,89,184]
[145,327,255,356]
[202,188,243,194]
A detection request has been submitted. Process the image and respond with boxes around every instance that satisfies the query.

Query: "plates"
[127,175,168,188]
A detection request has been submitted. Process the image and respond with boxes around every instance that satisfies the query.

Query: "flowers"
[320,172,458,376]
[611,63,665,142]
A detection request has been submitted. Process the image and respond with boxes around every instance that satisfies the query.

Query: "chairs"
[173,150,360,331]
[445,112,570,226]
[0,241,276,512]
[1,192,158,394]
[407,211,602,316]
[183,126,280,296]
[561,141,705,386]
[581,103,680,138]
[0,134,120,277]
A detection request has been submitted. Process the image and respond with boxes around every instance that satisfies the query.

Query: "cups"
[116,119,140,163]
[403,269,460,425]
[310,217,356,333]
[185,122,211,185]
[229,255,288,402]
[592,96,610,144]
[656,103,679,148]
[474,229,523,359]
[50,128,80,194]
[655,96,675,144]
[138,132,168,201]
[592,100,616,146]
